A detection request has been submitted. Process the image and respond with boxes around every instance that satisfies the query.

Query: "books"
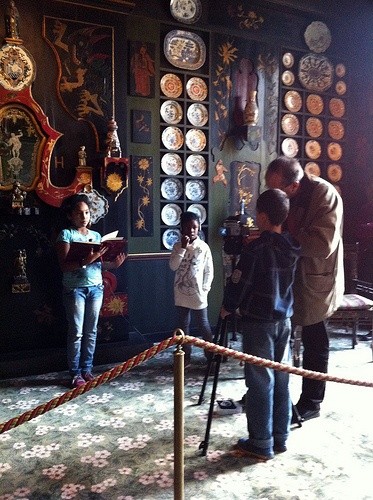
[67,229,129,263]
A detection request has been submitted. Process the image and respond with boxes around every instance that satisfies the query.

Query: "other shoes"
[272,439,287,451]
[237,438,274,459]
[290,404,320,425]
[83,373,94,382]
[71,375,86,388]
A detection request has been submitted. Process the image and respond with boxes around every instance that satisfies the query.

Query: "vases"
[242,90,259,127]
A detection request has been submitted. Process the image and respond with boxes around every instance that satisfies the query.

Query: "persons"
[164,211,216,369]
[243,156,346,425]
[54,194,130,389]
[219,187,302,460]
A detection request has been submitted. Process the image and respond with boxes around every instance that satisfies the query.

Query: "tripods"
[195,256,303,458]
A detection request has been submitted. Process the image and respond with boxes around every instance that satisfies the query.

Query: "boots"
[203,339,220,375]
[170,342,192,371]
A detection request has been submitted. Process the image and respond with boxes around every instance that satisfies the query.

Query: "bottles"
[242,91,260,127]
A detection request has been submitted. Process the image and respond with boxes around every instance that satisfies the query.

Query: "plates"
[185,76,209,101]
[187,104,209,127]
[284,89,302,113]
[161,204,207,252]
[335,65,348,96]
[185,179,207,200]
[161,126,184,151]
[169,0,202,24]
[160,178,184,201]
[163,29,207,70]
[185,155,207,177]
[282,53,296,86]
[160,153,183,176]
[300,55,336,91]
[305,21,333,53]
[306,94,325,115]
[160,73,183,98]
[159,101,183,124]
[329,98,347,118]
[281,114,345,182]
[185,129,207,151]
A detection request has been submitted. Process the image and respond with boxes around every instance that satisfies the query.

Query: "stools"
[291,293,373,365]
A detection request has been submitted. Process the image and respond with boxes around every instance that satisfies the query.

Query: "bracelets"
[79,258,86,269]
